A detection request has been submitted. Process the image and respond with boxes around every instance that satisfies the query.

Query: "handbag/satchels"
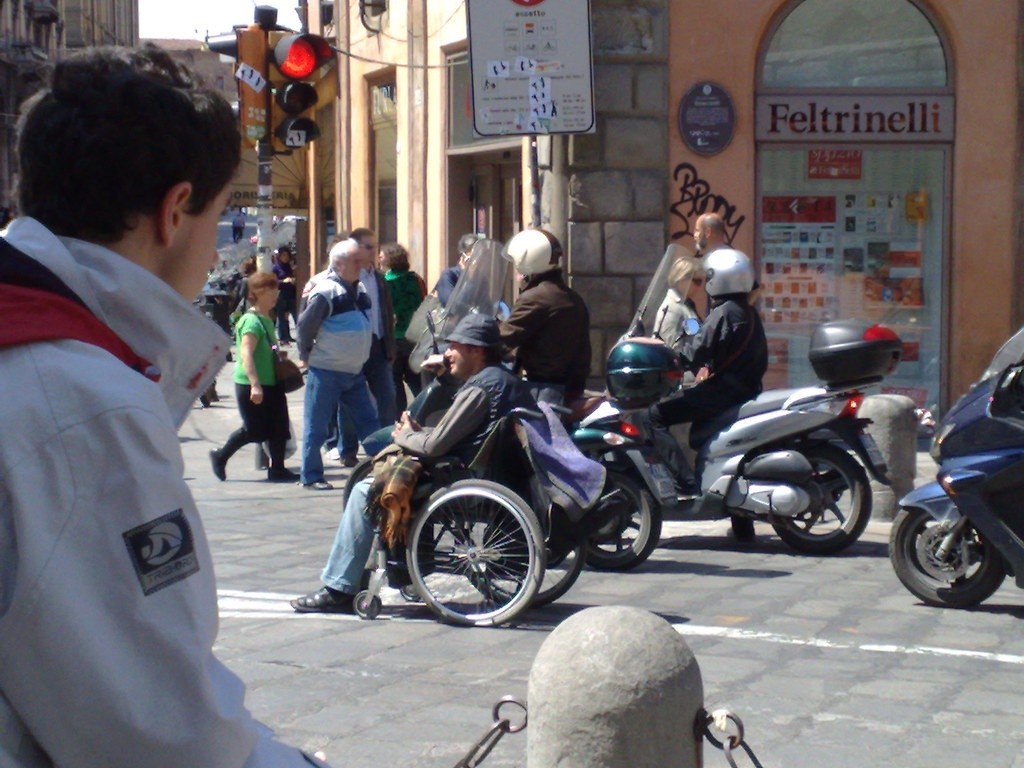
[408,305,462,374]
[274,358,305,394]
[403,276,454,348]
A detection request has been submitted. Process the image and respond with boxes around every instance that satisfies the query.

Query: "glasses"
[692,277,703,286]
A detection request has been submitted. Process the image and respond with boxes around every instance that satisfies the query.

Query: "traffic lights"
[206,31,241,119]
[275,30,336,149]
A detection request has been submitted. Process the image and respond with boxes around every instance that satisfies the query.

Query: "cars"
[218,221,259,249]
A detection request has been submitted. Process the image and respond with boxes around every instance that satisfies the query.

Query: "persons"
[199,209,591,489]
[0,207,17,229]
[0,40,333,768]
[639,212,769,544]
[290,313,532,614]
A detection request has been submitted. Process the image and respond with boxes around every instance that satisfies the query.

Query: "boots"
[285,320,296,341]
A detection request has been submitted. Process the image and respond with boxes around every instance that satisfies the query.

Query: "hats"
[445,312,502,349]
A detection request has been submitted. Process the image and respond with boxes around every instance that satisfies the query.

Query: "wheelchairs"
[352,398,595,630]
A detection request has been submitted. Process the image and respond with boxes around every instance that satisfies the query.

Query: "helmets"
[702,248,756,296]
[500,228,565,276]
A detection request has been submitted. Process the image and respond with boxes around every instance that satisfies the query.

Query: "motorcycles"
[344,225,662,573]
[569,317,900,556]
[888,325,1024,613]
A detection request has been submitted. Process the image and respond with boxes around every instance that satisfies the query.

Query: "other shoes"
[289,586,357,614]
[268,467,300,483]
[199,394,210,408]
[325,447,340,460]
[398,581,422,602]
[344,458,359,469]
[304,479,333,491]
[209,447,226,481]
[280,341,292,348]
